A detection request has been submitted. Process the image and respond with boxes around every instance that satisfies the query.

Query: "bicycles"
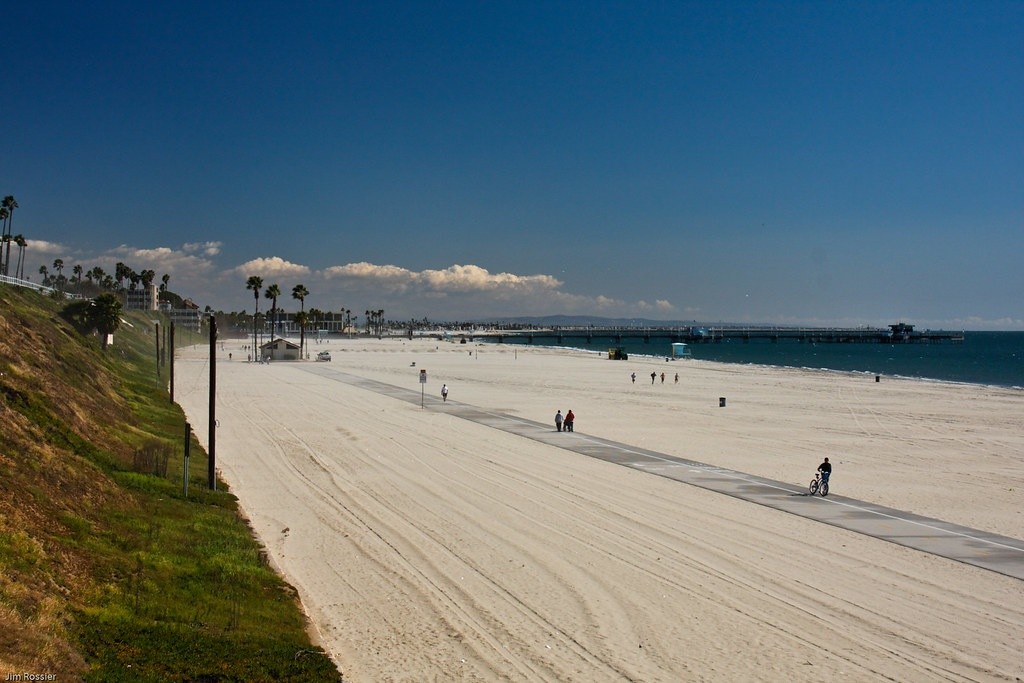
[809,471,830,497]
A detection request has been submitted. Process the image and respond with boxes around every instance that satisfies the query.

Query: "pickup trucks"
[317,352,332,362]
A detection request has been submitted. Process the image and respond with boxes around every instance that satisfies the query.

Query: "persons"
[441,384,448,402]
[228,344,271,364]
[555,410,563,431]
[650,372,656,383]
[675,373,678,383]
[631,373,636,383]
[660,373,664,382]
[817,458,832,493]
[566,410,574,432]
